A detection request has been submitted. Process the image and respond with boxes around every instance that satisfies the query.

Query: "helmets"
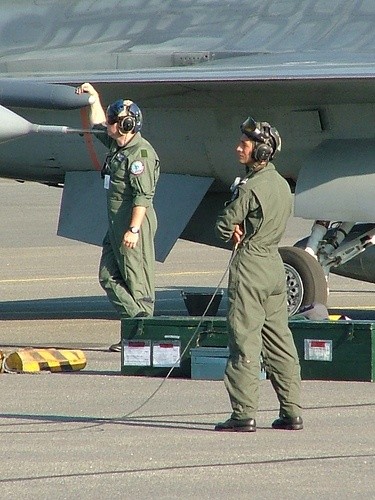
[106,98,142,133]
[246,121,281,160]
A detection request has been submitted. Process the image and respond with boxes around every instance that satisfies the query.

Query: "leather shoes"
[215,418,256,432]
[136,312,148,317]
[272,416,303,430]
[109,341,122,351]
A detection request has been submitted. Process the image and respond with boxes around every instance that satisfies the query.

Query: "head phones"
[120,102,139,134]
[253,125,270,162]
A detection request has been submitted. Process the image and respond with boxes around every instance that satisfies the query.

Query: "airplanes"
[1,0,374,322]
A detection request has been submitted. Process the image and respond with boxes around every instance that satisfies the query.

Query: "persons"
[75,83,160,351]
[216,117,304,431]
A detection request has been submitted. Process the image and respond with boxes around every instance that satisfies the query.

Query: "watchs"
[128,226,139,233]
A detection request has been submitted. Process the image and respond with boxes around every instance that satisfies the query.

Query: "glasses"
[240,116,257,134]
[108,115,120,125]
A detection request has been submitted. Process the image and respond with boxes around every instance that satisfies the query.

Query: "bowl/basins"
[181,290,224,316]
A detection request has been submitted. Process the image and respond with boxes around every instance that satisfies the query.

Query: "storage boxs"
[120,315,375,382]
